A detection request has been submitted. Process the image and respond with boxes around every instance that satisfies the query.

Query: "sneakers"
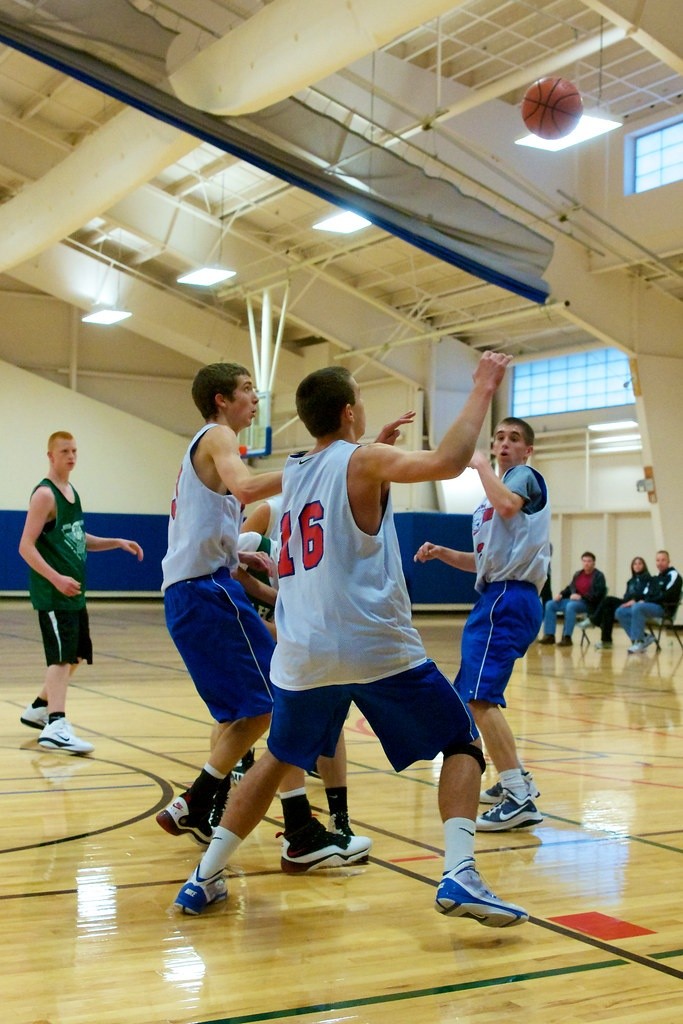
[478,772,541,804]
[275,817,374,875]
[328,811,370,863]
[436,859,530,928]
[627,639,647,654]
[641,633,655,647]
[37,717,94,753]
[231,748,256,783]
[475,788,543,832]
[172,864,227,916]
[155,787,217,850]
[20,705,51,730]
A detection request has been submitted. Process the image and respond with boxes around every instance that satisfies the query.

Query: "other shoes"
[557,635,572,646]
[576,617,594,629]
[538,634,555,645]
[595,640,612,649]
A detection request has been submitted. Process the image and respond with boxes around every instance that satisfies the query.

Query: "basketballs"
[521,76,584,140]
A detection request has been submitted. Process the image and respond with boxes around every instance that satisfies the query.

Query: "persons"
[413,416,554,833]
[615,550,683,653]
[156,363,374,878]
[577,557,651,649]
[538,550,607,646]
[154,347,554,928]
[19,431,145,751]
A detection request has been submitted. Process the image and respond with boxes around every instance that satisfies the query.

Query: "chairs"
[575,586,683,653]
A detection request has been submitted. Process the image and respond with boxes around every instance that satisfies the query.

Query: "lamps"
[175,166,237,288]
[80,228,131,324]
[637,478,655,493]
[312,205,370,233]
[514,12,622,151]
